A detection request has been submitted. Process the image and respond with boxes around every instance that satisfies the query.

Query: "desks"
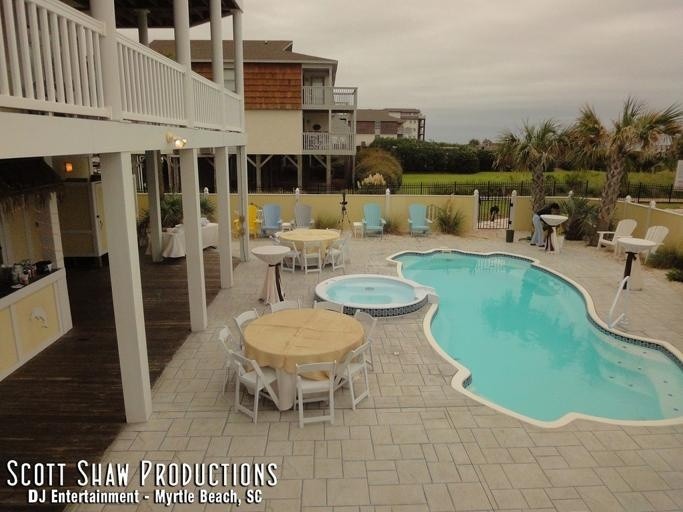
[145,223,218,264]
[541,215,569,255]
[618,238,655,290]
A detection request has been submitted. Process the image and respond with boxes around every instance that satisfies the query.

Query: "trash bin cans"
[506,230,514,242]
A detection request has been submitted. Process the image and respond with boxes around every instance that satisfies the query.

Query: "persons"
[528,202,560,247]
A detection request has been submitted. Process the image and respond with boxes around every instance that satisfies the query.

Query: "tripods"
[337,205,355,238]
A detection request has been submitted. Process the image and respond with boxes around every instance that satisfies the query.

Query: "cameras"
[339,201,347,205]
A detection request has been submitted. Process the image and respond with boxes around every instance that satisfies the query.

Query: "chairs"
[408,202,433,239]
[217,202,386,429]
[616,225,668,264]
[596,219,636,253]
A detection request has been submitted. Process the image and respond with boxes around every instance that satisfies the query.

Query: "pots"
[35,261,52,273]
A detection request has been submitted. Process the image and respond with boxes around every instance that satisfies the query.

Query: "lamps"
[165,133,189,151]
